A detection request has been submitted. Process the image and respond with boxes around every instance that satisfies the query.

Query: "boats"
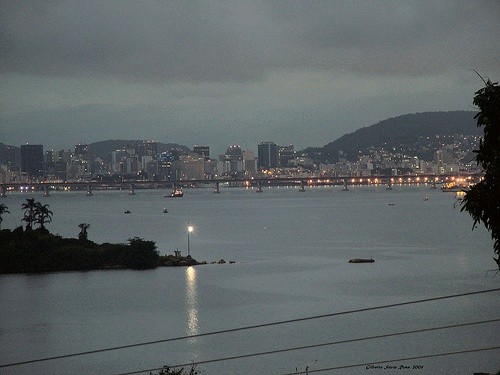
[163,208,168,213]
[164,190,183,197]
[349,257,375,263]
[424,197,429,201]
[125,210,131,214]
[455,191,467,200]
[441,181,473,191]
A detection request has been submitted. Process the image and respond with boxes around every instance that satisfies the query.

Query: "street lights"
[187,225,193,255]
